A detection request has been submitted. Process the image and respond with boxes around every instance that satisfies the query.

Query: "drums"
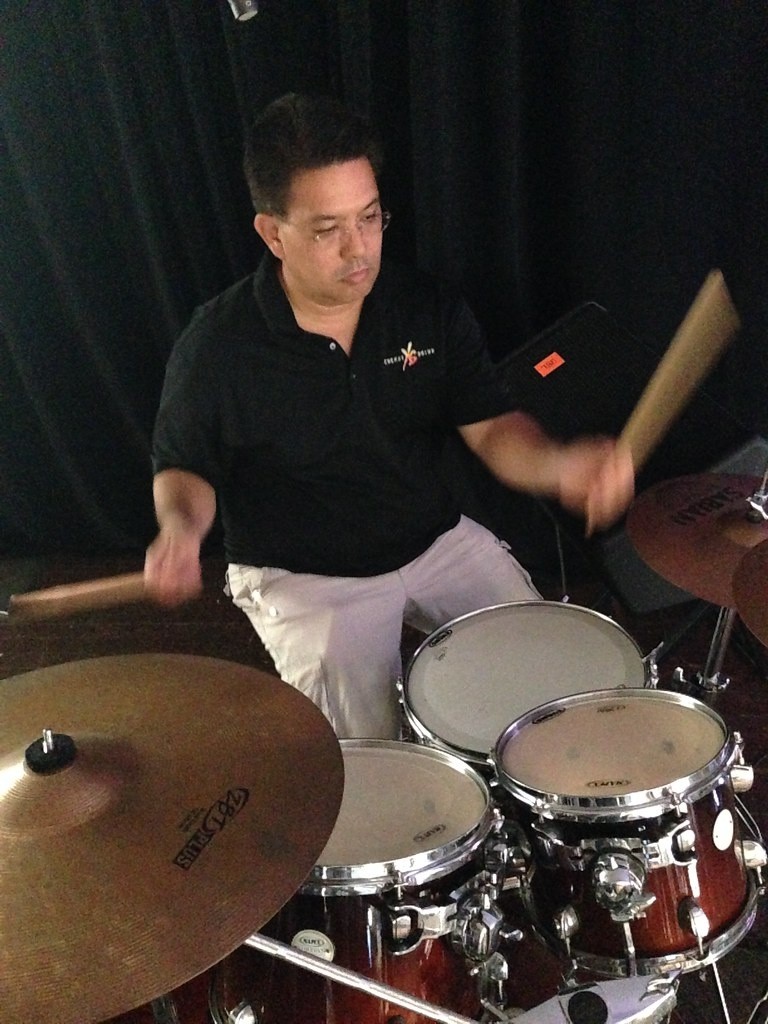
[480,873,678,1024]
[481,683,764,982]
[396,600,659,781]
[206,728,502,1023]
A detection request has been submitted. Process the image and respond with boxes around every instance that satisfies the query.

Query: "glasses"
[267,209,393,242]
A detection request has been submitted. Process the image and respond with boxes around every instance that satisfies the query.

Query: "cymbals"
[0,649,348,1023]
[733,538,767,644]
[624,470,768,609]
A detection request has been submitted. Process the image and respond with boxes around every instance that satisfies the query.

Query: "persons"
[144,97,613,739]
